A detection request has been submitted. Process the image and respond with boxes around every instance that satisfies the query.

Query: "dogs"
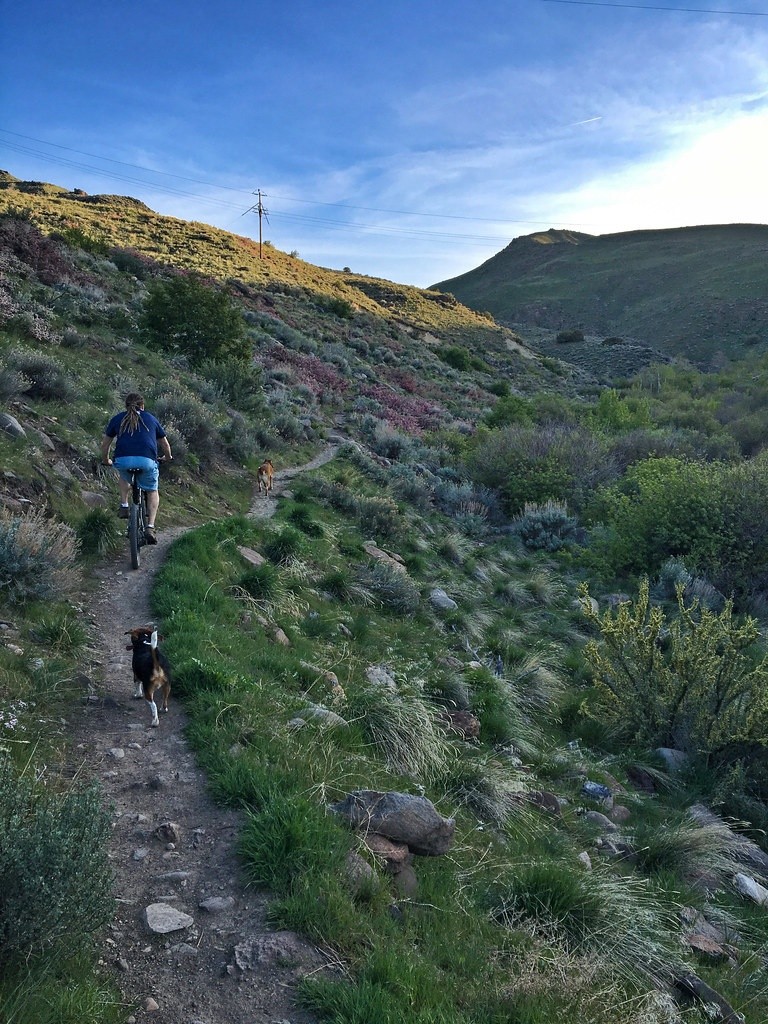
[124,628,171,727]
[257,459,274,496]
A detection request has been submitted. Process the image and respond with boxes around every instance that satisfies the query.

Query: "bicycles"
[105,455,172,570]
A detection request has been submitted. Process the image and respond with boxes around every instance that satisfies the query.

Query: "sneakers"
[117,505,129,519]
[145,527,158,545]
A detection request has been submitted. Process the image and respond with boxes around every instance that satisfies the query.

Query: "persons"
[103,393,172,545]
